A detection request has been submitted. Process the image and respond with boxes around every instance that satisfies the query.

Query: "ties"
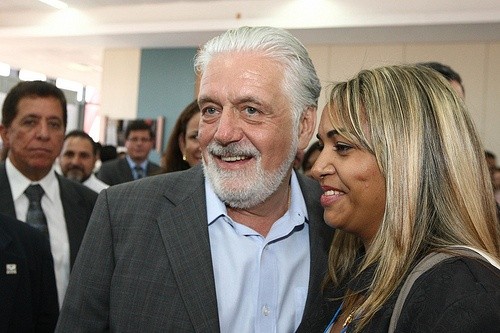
[135,165,144,180]
[23,184,61,333]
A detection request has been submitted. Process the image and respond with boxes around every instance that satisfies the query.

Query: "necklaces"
[342,302,359,329]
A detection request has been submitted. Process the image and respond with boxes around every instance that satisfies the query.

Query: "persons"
[0,79,99,333]
[296,63,500,333]
[53,26,365,333]
[1,214,60,333]
[55,97,207,194]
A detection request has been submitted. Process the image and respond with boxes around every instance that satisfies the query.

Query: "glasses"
[126,136,153,143]
